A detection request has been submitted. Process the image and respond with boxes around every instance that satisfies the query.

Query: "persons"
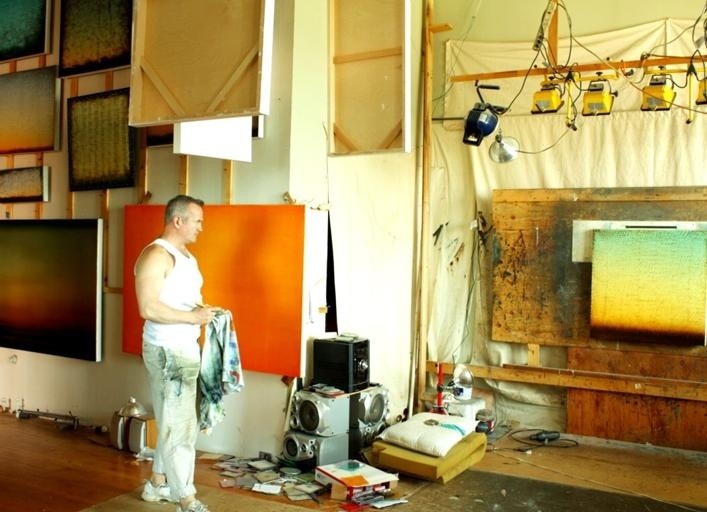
[132,195,222,511]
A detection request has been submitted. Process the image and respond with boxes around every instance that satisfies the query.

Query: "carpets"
[75,482,322,512]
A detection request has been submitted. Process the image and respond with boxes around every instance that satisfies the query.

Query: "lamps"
[461,0,706,167]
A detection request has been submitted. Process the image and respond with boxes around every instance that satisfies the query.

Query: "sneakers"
[176,499,210,512]
[140,479,179,503]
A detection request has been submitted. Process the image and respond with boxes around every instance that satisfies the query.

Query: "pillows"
[373,410,483,459]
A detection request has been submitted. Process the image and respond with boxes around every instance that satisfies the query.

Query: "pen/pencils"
[195,304,204,308]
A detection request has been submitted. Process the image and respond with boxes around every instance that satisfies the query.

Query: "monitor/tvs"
[0,217,103,364]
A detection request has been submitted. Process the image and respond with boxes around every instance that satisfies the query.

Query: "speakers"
[314,338,369,393]
[349,418,387,459]
[283,433,349,473]
[290,387,351,437]
[127,416,157,453]
[347,386,389,427]
[110,412,129,450]
